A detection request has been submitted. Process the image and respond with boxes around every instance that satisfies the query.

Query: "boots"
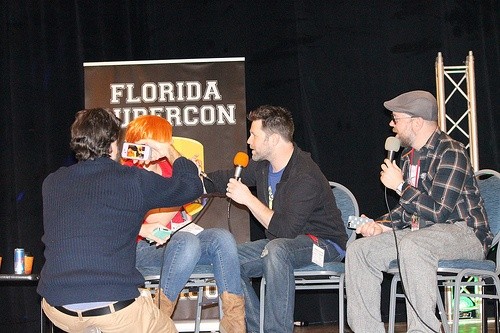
[153,290,178,318]
[219,291,248,333]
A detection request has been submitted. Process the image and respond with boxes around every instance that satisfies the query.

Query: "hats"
[384,91,438,121]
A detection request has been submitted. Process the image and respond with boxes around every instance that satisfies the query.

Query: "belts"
[55,298,135,317]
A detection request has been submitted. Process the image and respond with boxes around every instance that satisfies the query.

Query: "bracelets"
[398,181,405,191]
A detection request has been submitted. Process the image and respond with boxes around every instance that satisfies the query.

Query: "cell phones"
[347,216,374,229]
[122,142,150,161]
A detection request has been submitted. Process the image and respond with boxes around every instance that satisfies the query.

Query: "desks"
[0,273,54,333]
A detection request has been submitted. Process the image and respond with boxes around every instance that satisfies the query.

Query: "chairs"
[387,169,500,333]
[260,181,359,333]
[138,265,224,333]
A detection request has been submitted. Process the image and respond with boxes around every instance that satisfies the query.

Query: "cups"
[25,257,34,274]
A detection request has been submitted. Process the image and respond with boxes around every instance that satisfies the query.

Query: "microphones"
[385,136,401,163]
[200,171,213,182]
[233,152,248,181]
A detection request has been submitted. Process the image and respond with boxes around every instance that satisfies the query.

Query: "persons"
[202,105,348,333]
[344,91,494,333]
[121,115,246,333]
[36,108,204,333]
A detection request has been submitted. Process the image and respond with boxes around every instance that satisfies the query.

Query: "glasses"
[391,115,419,124]
